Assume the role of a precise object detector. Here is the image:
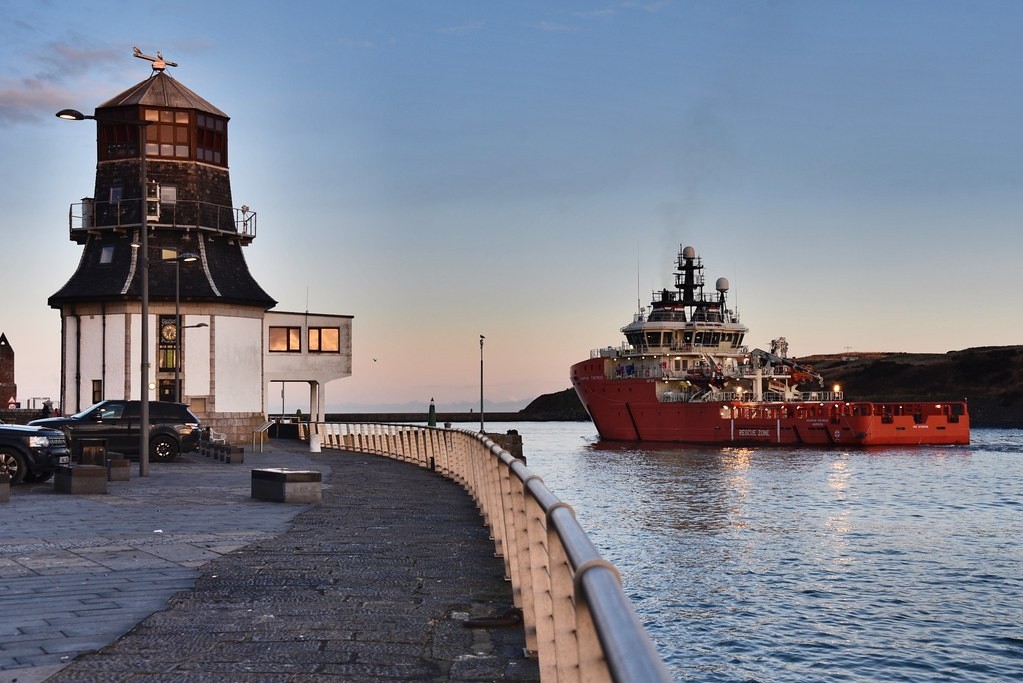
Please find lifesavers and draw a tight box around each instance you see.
[703,366,712,377]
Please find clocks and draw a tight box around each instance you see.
[162,324,176,341]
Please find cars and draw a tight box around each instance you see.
[0,418,73,484]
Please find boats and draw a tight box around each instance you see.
[571,243,971,449]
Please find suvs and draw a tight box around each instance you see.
[26,398,203,462]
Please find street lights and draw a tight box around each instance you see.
[54,105,152,476]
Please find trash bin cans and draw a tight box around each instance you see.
[76,437,109,467]
